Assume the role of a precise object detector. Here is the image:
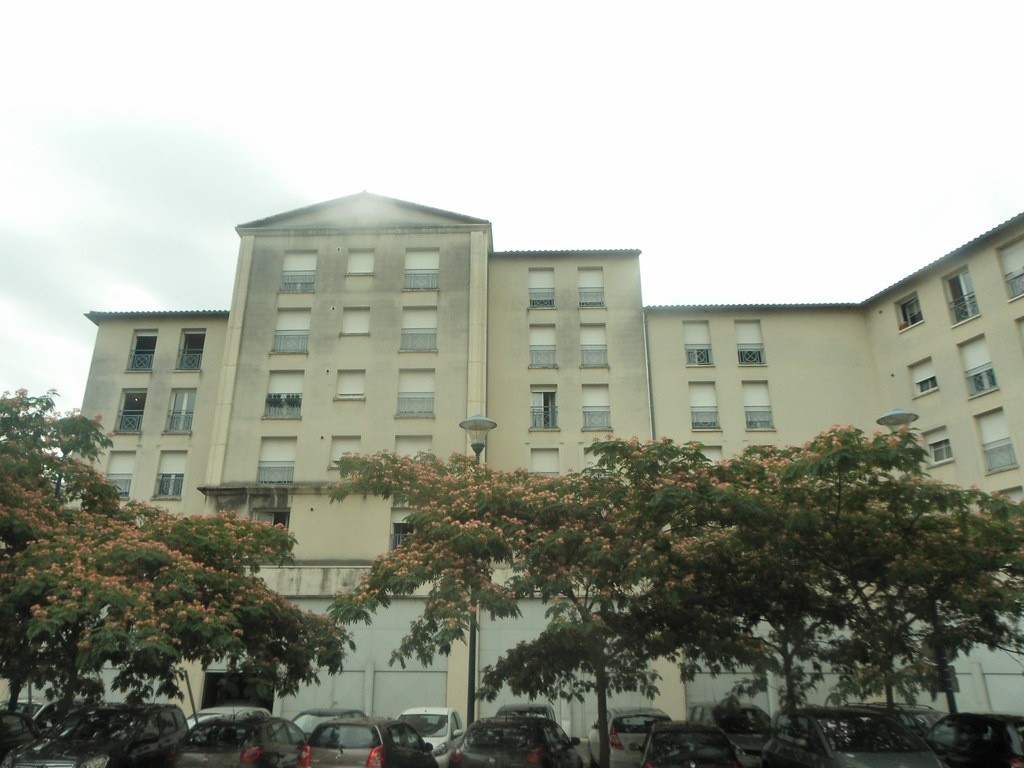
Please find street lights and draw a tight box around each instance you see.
[458,413,498,729]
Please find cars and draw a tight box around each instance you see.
[1,700,1024,768]
